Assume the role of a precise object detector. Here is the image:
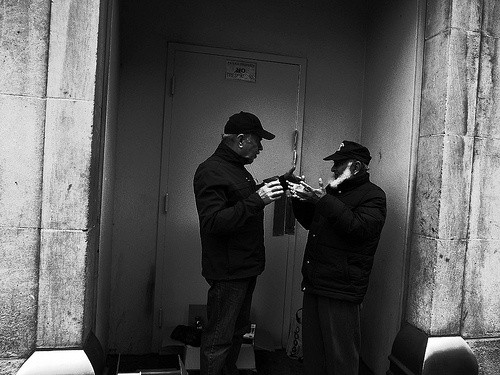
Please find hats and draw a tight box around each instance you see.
[224,111,275,140]
[323,141,372,165]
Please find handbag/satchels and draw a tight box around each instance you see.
[170,325,202,347]
[286,308,303,360]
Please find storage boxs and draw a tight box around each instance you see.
[161,304,256,370]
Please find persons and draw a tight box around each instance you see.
[286,140,387,375]
[193,110,306,375]
